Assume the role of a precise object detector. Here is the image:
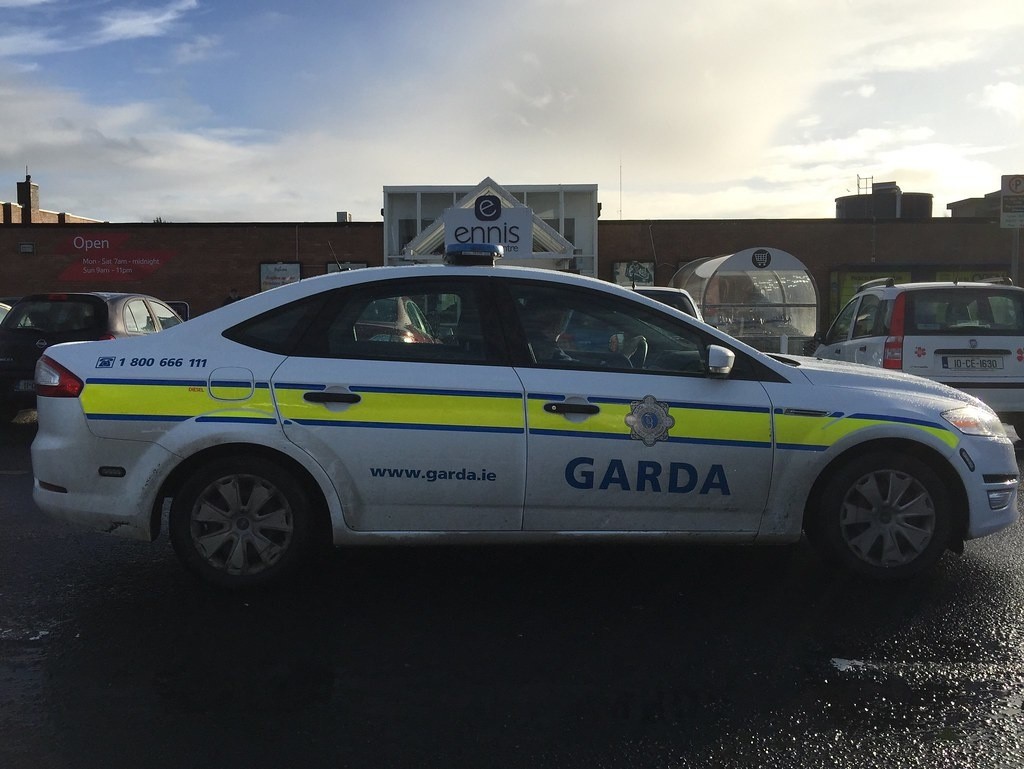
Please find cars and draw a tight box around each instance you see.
[816,274,1024,451]
[0,303,34,331]
[30,241,1019,597]
[353,296,455,346]
[0,290,190,437]
[543,285,722,377]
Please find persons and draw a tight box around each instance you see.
[525,293,647,363]
